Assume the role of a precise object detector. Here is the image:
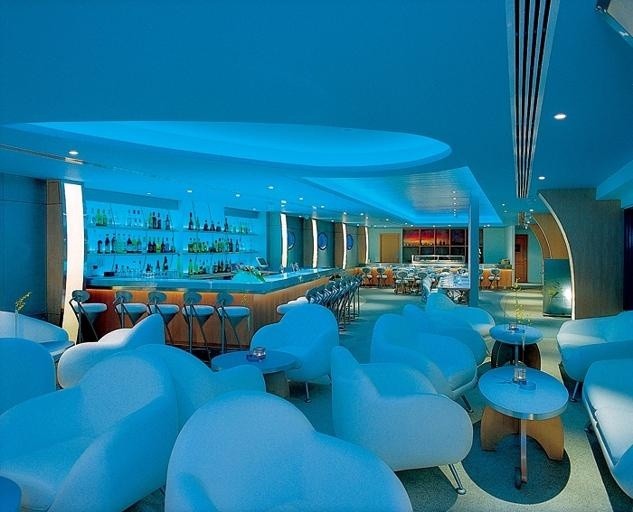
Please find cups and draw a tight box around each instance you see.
[513,367,526,384]
[508,322,516,330]
[252,347,266,358]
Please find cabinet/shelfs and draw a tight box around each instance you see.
[81,196,180,280]
[182,209,259,277]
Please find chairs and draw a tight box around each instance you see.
[357,262,502,302]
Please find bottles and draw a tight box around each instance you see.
[176,253,182,280]
[187,209,252,275]
[89,206,174,277]
